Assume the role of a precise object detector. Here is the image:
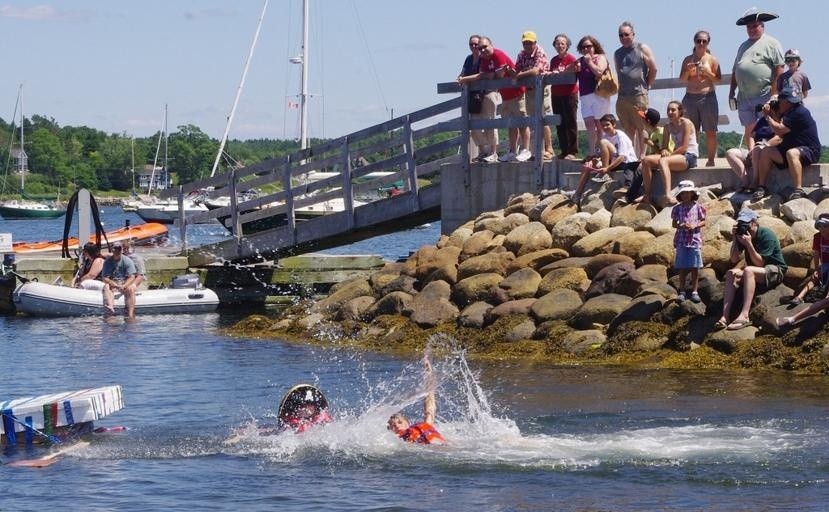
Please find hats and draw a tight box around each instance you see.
[638,108,661,124]
[521,31,537,44]
[736,7,780,26]
[736,209,759,223]
[814,213,829,230]
[670,179,700,202]
[776,87,802,105]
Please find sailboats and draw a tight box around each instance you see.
[205,0,393,237]
[0,83,66,220]
[137,99,284,224]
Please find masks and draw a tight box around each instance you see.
[783,49,801,60]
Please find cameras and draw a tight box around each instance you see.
[755,101,776,112]
[734,224,751,238]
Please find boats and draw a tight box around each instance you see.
[14,281,218,316]
[1,222,169,260]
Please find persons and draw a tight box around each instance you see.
[74,242,106,291]
[563,36,612,162]
[502,32,556,159]
[729,7,786,152]
[120,240,148,291]
[753,88,821,200]
[387,359,448,446]
[613,22,658,161]
[551,35,580,160]
[715,209,788,330]
[572,114,641,204]
[478,36,532,162]
[680,31,722,167]
[672,180,707,303]
[611,109,676,206]
[286,404,332,435]
[790,214,829,304]
[633,101,699,206]
[456,35,501,163]
[777,50,812,107]
[776,292,829,327]
[726,100,783,193]
[102,245,137,324]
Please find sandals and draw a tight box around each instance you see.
[564,153,578,159]
[750,185,770,200]
[544,149,556,161]
[676,290,687,301]
[689,291,702,303]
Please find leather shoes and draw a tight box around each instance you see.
[782,298,802,306]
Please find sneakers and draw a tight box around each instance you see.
[472,152,487,163]
[590,172,614,183]
[617,196,628,204]
[775,316,795,328]
[496,149,517,162]
[513,148,533,162]
[612,186,629,197]
[571,196,580,204]
[634,194,651,203]
[664,194,677,206]
[483,151,499,162]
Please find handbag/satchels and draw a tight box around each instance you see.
[594,54,618,99]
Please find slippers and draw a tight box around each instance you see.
[713,319,730,331]
[726,319,753,330]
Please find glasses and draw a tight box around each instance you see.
[580,45,594,50]
[696,39,708,44]
[523,42,534,46]
[785,58,797,63]
[478,45,489,50]
[468,43,479,46]
[619,32,632,37]
[746,23,761,28]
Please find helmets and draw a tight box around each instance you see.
[110,240,123,256]
